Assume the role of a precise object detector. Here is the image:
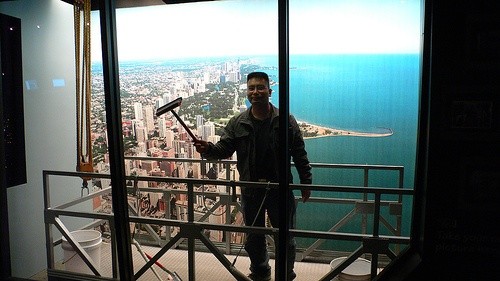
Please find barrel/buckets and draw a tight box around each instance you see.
[330,256,371,281]
[62,230,102,276]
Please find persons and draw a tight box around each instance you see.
[191,72,314,281]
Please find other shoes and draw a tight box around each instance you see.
[247,275,271,281]
[287,272,296,280]
